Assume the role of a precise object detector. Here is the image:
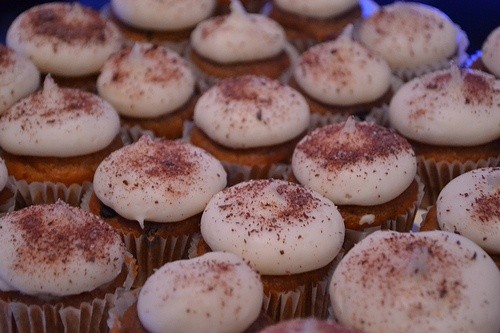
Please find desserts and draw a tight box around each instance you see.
[0,0,500,333]
